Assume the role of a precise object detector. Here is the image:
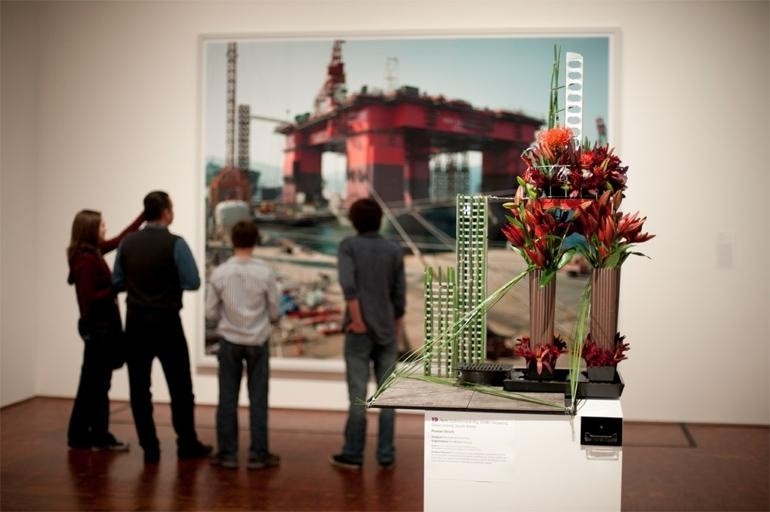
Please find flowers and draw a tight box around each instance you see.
[504,128,656,269]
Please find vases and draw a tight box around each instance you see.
[503,268,625,404]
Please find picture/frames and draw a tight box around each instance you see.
[194,29,621,373]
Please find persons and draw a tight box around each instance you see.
[204,220,283,471]
[107,190,213,465]
[328,198,407,473]
[65,209,147,449]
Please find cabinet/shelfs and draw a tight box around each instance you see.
[369,360,623,512]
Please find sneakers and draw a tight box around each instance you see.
[206,448,240,470]
[142,435,213,464]
[92,441,131,454]
[327,454,396,471]
[245,444,281,468]
